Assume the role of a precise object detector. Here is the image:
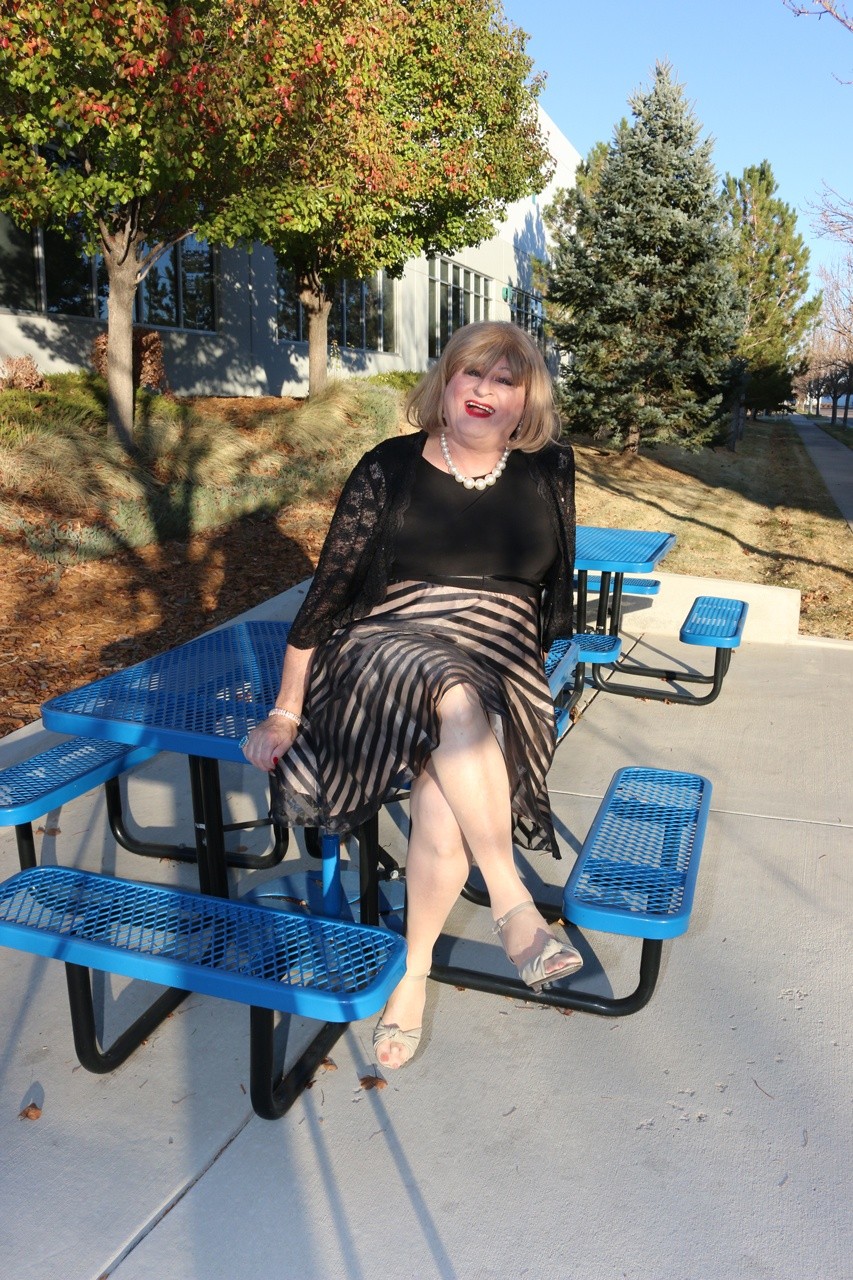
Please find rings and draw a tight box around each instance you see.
[239,736,249,749]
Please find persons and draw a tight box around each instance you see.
[239,321,586,1076]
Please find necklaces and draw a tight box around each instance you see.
[438,427,512,490]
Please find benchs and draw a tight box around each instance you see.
[0,864,408,1120]
[0,735,162,870]
[563,633,626,714]
[679,595,751,706]
[572,573,662,631]
[559,766,716,1018]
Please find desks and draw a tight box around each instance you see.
[37,617,582,973]
[561,524,677,689]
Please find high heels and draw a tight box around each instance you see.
[372,970,431,1070]
[491,901,584,988]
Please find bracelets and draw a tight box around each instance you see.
[268,709,303,727]
